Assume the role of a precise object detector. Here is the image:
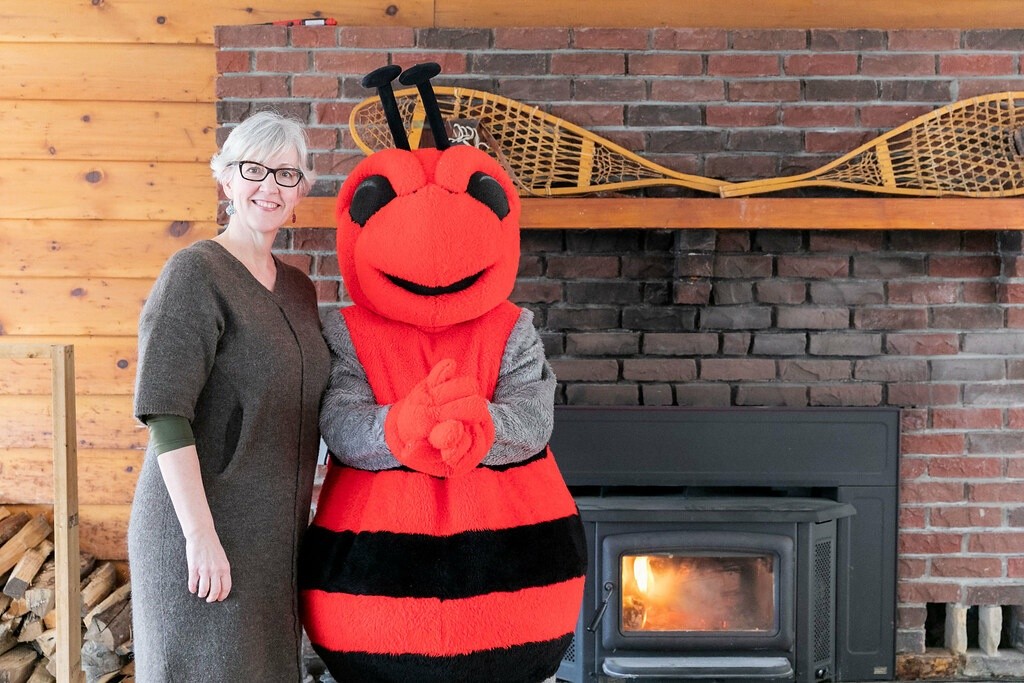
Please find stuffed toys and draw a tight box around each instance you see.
[297,58,583,683]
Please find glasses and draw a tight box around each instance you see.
[227,161,304,188]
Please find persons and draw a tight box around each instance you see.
[115,110,339,683]
